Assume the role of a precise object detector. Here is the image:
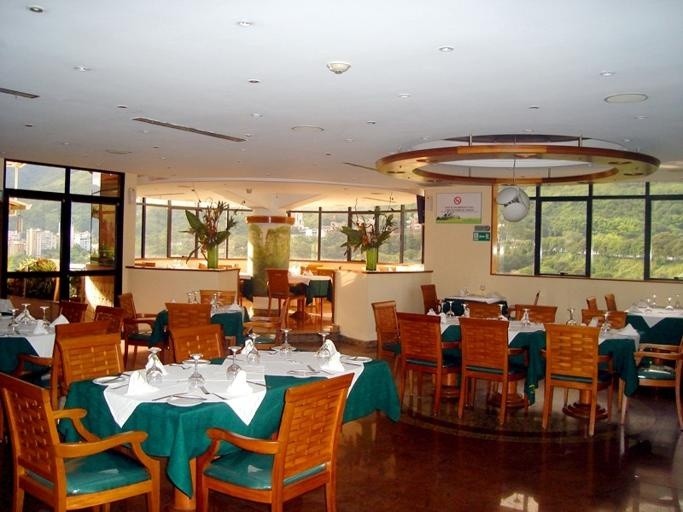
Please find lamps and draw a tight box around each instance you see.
[496,159,530,222]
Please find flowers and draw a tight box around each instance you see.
[178,190,246,264]
[340,195,399,256]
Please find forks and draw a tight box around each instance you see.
[246,382,271,389]
[201,386,227,400]
[306,364,332,377]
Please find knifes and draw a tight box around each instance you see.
[153,393,185,401]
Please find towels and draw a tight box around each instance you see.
[316,352,348,374]
[238,340,255,357]
[618,323,638,338]
[146,353,167,374]
[123,372,159,398]
[222,370,256,398]
[49,312,68,330]
[29,320,44,335]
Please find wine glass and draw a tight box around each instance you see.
[497,304,504,320]
[643,289,681,313]
[445,301,455,324]
[226,346,242,381]
[520,309,531,331]
[319,332,330,365]
[279,328,292,360]
[600,314,610,336]
[146,348,162,386]
[187,290,225,314]
[248,334,260,365]
[189,354,205,392]
[438,302,445,323]
[8,308,20,336]
[21,304,32,331]
[461,304,469,318]
[40,306,50,333]
[565,309,577,326]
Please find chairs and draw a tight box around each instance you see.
[200,289,236,306]
[507,291,540,318]
[458,318,528,426]
[582,309,628,413]
[28,299,60,323]
[605,294,617,310]
[170,324,225,363]
[300,264,322,313]
[586,296,598,310]
[494,304,558,399]
[119,293,156,368]
[59,300,88,323]
[55,332,124,409]
[93,305,125,329]
[621,337,683,430]
[465,303,501,400]
[8,295,30,316]
[196,372,354,512]
[165,303,213,329]
[371,301,404,378]
[396,312,462,417]
[421,284,441,315]
[0,373,160,512]
[542,322,614,437]
[317,267,338,323]
[243,298,289,350]
[17,319,111,425]
[265,268,305,329]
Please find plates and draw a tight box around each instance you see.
[287,369,312,378]
[184,359,211,366]
[272,345,297,351]
[168,396,203,407]
[351,356,374,363]
[92,376,126,387]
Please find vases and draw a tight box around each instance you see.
[366,247,377,270]
[208,243,218,268]
